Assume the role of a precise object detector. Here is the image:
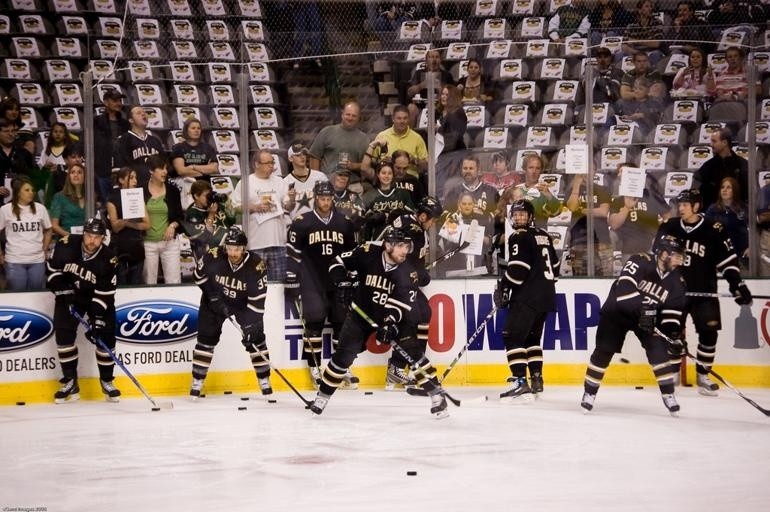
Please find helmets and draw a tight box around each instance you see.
[511,199,534,212]
[677,188,702,203]
[314,181,335,195]
[653,232,685,253]
[84,218,107,236]
[383,226,413,242]
[225,228,247,246]
[418,196,443,218]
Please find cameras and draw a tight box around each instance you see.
[207,192,228,206]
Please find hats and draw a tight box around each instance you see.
[103,89,127,99]
[596,47,612,56]
[287,144,310,157]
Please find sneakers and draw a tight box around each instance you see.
[581,391,596,410]
[500,377,531,397]
[310,366,322,383]
[258,375,272,395]
[661,393,680,411]
[697,372,719,390]
[191,376,204,396]
[100,376,120,397]
[531,376,543,393]
[310,392,330,414]
[344,369,359,383]
[431,391,447,413]
[386,364,411,381]
[55,376,79,398]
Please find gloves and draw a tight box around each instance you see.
[336,284,361,312]
[241,323,261,347]
[637,313,655,337]
[666,332,688,357]
[85,313,107,344]
[494,285,511,309]
[284,282,304,304]
[730,276,752,304]
[64,294,78,321]
[375,320,400,344]
[207,290,231,317]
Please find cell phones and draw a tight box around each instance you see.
[538,179,544,185]
[289,181,296,191]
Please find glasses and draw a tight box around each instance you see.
[261,161,275,165]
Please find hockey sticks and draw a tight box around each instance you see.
[65,303,173,410]
[352,300,486,409]
[293,301,360,384]
[654,326,770,417]
[224,310,315,406]
[407,304,499,396]
[426,219,478,269]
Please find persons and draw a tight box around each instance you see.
[652,190,752,397]
[455,58,498,115]
[386,196,444,392]
[0,91,770,291]
[546,1,769,91]
[579,234,689,418]
[309,227,451,420]
[407,51,454,130]
[291,0,327,70]
[418,1,458,27]
[434,83,471,154]
[188,230,277,395]
[285,183,359,390]
[492,199,561,399]
[370,1,433,32]
[46,218,124,403]
